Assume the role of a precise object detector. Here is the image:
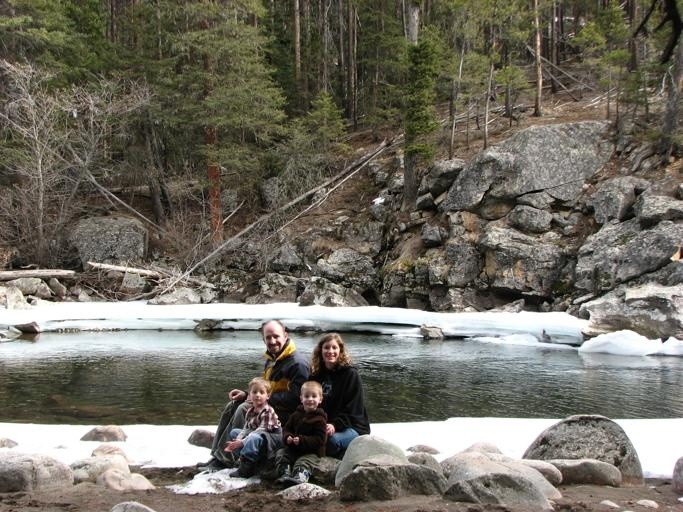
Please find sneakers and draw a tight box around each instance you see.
[197,458,217,467]
[198,460,224,472]
[287,466,310,484]
[276,463,291,483]
[230,461,255,478]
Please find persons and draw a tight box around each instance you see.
[311,333,371,459]
[275,380,327,484]
[197,318,310,473]
[224,377,283,478]
